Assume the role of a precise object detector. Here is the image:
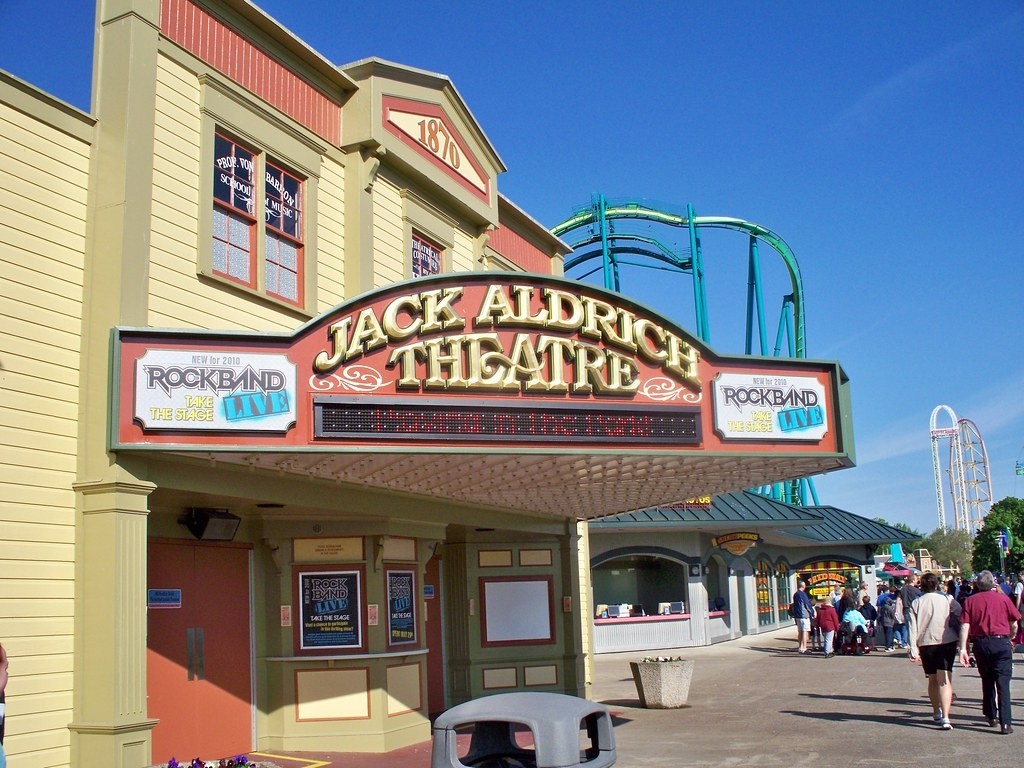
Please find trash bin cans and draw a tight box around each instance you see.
[431,690,617,768]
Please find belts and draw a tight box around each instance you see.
[975,635,1006,640]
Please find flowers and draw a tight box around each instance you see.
[640,655,684,662]
[159,754,256,768]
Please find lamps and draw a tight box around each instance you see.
[865,566,872,574]
[727,567,734,577]
[702,566,709,576]
[175,507,241,541]
[753,567,758,577]
[774,570,778,577]
[688,565,700,577]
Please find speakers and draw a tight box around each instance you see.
[185,510,242,541]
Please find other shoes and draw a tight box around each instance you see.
[799,648,812,655]
[941,720,952,729]
[932,710,942,723]
[825,653,834,658]
[989,717,999,727]
[884,648,894,652]
[1000,723,1013,734]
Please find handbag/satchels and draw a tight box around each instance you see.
[947,612,960,632]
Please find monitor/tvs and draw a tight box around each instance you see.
[715,598,725,607]
[657,602,683,614]
[596,605,643,617]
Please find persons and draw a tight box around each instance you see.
[957,570,1022,735]
[905,573,964,729]
[792,568,1023,659]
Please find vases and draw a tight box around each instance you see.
[147,759,280,768]
[629,658,695,709]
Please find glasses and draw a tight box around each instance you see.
[1018,574,1024,578]
